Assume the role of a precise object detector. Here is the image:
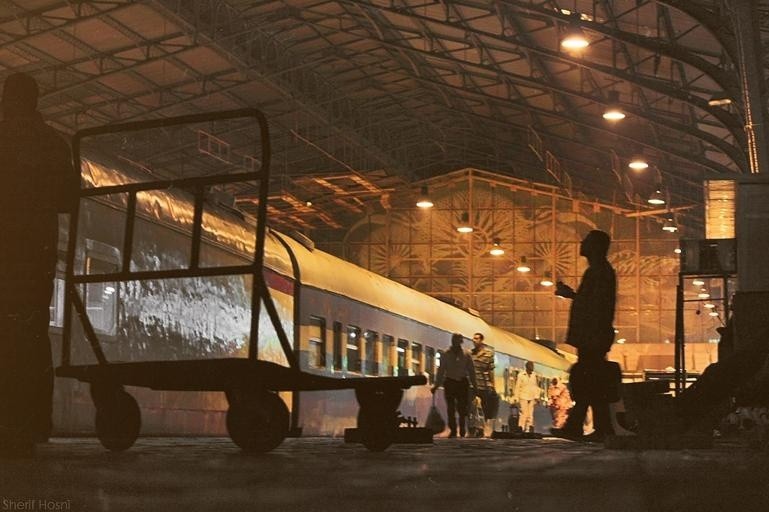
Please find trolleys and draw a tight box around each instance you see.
[54,106,427,455]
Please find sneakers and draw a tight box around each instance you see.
[549,427,585,443]
[585,429,617,444]
[425,406,445,434]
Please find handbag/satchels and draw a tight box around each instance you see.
[569,360,621,403]
[487,392,500,419]
[468,396,485,431]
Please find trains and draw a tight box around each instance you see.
[49,154,593,438]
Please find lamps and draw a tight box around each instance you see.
[646,179,677,231]
[414,154,554,288]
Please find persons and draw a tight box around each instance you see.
[549,377,575,429]
[466,332,495,439]
[547,230,624,445]
[429,332,478,438]
[513,360,540,434]
